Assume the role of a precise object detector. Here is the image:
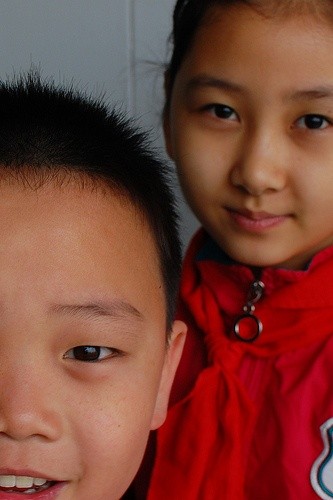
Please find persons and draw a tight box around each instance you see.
[119,0,333,500]
[0,74,187,500]
